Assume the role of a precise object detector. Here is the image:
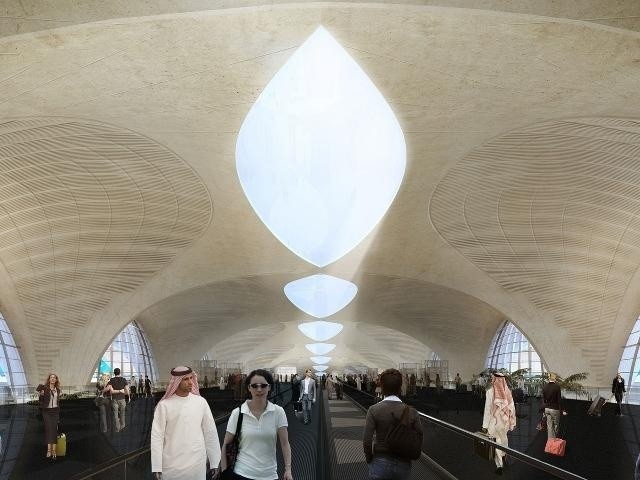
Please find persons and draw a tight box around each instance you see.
[129,375,137,401]
[471,371,477,398]
[517,374,524,391]
[435,373,440,388]
[424,375,431,391]
[221,370,294,480]
[363,368,424,479]
[137,374,144,400]
[148,364,222,479]
[482,372,517,476]
[95,372,125,433]
[478,373,485,398]
[455,372,462,392]
[202,375,209,390]
[142,375,153,400]
[611,369,626,416]
[39,373,61,459]
[97,368,131,433]
[542,372,569,442]
[299,368,317,426]
[220,372,417,415]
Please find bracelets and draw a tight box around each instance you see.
[284,463,291,468]
